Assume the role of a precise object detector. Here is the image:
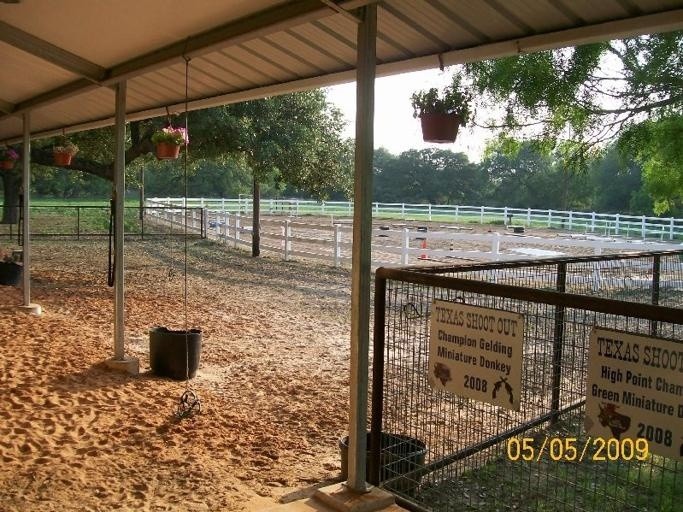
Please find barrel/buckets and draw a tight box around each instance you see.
[149,326,204,378]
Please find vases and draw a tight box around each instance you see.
[0,160,14,169]
[51,153,72,166]
[156,143,180,159]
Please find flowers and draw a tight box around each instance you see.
[0,146,19,162]
[151,125,189,147]
[53,142,80,157]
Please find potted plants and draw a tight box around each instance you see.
[411,75,474,143]
